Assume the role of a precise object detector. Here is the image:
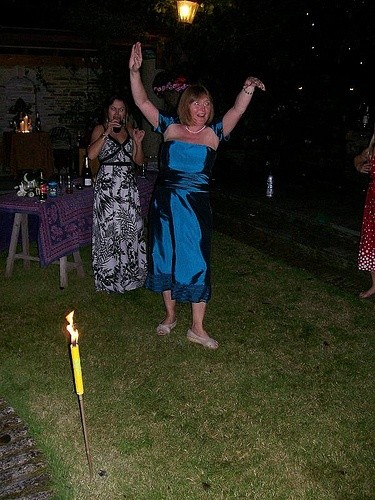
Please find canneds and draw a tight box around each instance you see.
[48,182,58,198]
[28,187,35,198]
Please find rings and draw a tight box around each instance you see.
[259,81,262,83]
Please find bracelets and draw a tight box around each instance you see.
[244,89,253,95]
[100,132,107,140]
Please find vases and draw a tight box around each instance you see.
[162,91,182,108]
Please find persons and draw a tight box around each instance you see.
[87,95,148,292]
[129,42,265,350]
[18,112,33,133]
[353,131,375,299]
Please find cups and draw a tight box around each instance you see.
[48,183,58,198]
[56,174,65,196]
[64,175,74,194]
[113,114,121,133]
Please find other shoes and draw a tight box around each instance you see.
[187,328,220,350]
[156,316,178,335]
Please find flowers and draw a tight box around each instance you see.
[152,81,191,95]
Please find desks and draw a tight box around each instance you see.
[0,170,159,290]
[3,132,52,178]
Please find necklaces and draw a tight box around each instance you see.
[184,125,206,134]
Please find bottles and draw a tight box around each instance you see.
[76,130,82,147]
[264,172,275,201]
[358,102,370,137]
[83,156,92,187]
[141,162,147,178]
[34,111,41,132]
[38,171,47,201]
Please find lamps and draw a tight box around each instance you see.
[176,0,200,25]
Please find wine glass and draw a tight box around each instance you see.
[9,120,17,135]
